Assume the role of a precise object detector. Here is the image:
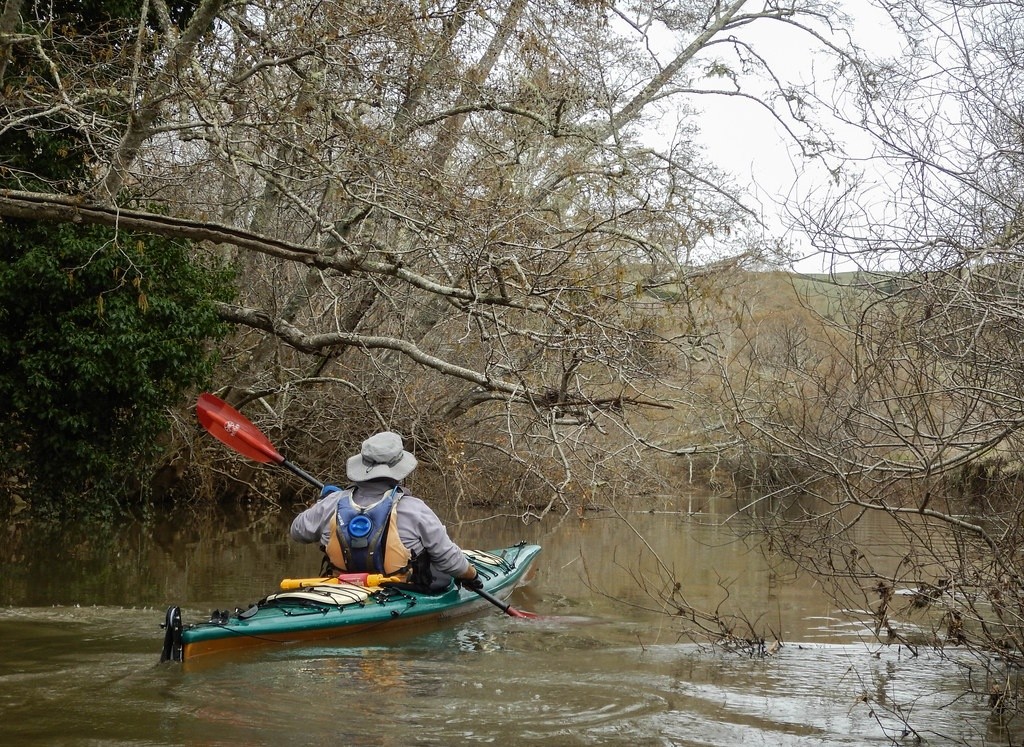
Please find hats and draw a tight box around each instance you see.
[347,431,418,482]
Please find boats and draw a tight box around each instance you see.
[160,539,542,664]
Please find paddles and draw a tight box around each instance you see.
[195,392,535,617]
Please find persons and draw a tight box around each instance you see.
[291,432,484,595]
